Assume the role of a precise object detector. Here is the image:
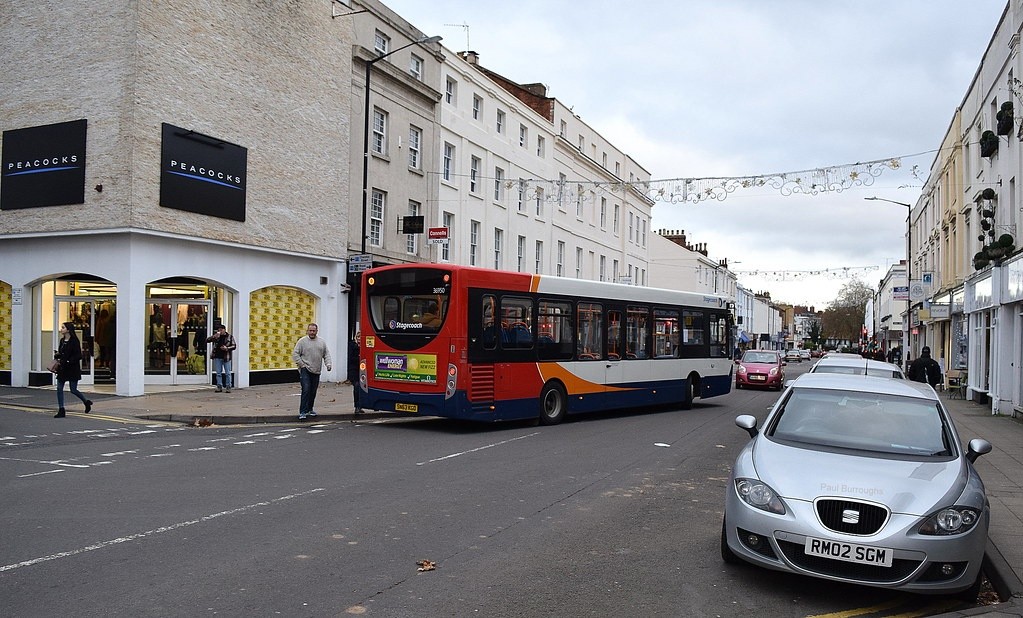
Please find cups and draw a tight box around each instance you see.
[217,332,220,337]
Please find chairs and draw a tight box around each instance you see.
[946,369,970,400]
[483,319,637,361]
[747,355,777,362]
[800,398,929,439]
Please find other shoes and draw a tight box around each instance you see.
[215,386,222,393]
[226,385,232,393]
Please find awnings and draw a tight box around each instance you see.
[741,331,753,342]
[867,340,873,348]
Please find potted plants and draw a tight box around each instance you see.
[995,102,1013,136]
[979,131,999,158]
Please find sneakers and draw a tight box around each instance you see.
[306,410,317,416]
[299,412,306,420]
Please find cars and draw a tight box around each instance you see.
[721,372,994,602]
[735,350,786,392]
[786,350,802,362]
[776,350,788,362]
[810,351,820,358]
[811,355,907,381]
[799,350,811,361]
[822,352,862,359]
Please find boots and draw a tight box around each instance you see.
[84,400,93,413]
[54,407,66,418]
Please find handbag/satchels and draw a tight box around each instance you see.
[47,358,61,374]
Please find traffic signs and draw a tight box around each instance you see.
[349,262,373,273]
[349,253,373,264]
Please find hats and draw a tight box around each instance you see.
[922,346,930,354]
[218,325,225,329]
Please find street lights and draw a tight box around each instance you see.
[362,33,444,254]
[864,288,876,360]
[863,195,912,377]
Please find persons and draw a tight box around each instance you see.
[865,348,894,363]
[150,313,167,367]
[422,303,441,327]
[908,346,941,390]
[80,310,111,368]
[630,342,639,359]
[54,323,93,418]
[293,324,332,419]
[348,332,364,414]
[206,325,236,393]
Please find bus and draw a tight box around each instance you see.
[359,263,739,427]
